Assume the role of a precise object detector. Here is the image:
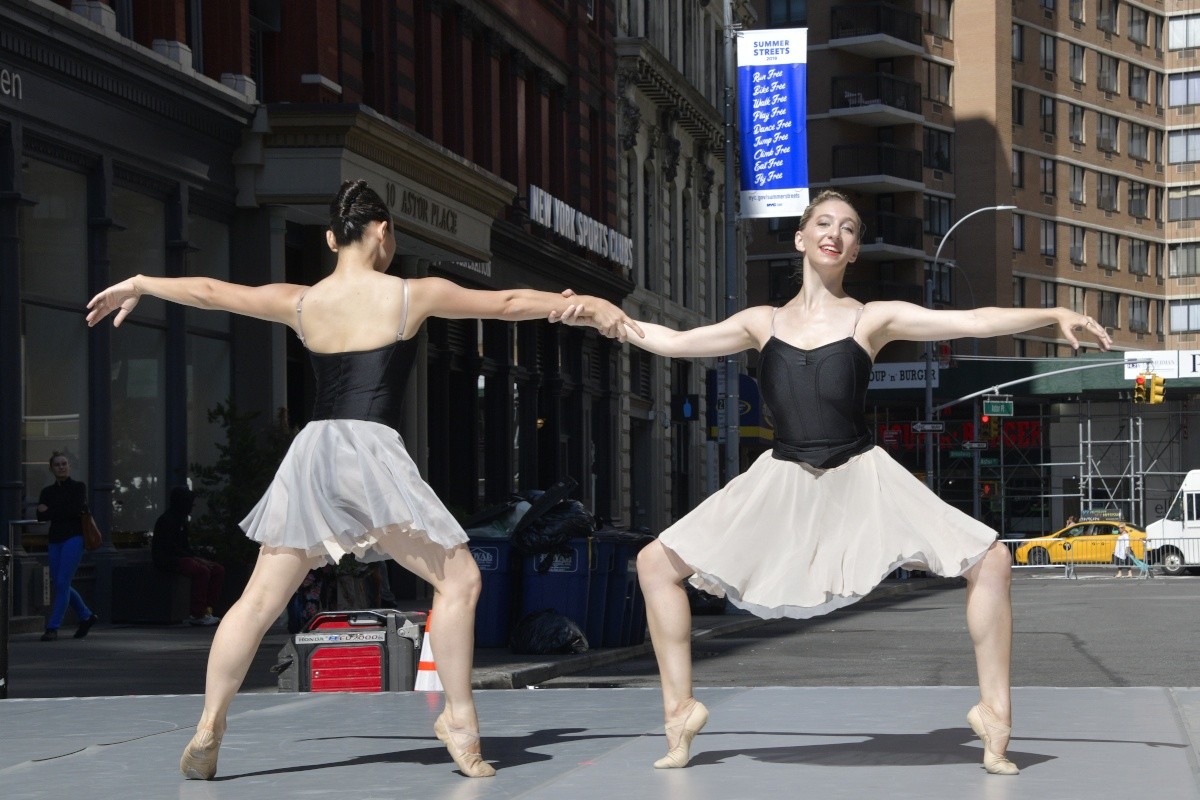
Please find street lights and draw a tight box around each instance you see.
[943,262,981,521]
[926,205,1019,487]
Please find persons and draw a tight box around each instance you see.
[547,191,1112,777]
[1065,516,1078,528]
[152,486,224,625]
[36,451,103,641]
[86,178,646,780]
[1114,521,1134,577]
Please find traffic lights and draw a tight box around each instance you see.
[984,481,997,495]
[990,416,1000,442]
[981,414,990,443]
[1150,374,1166,405]
[1134,375,1147,404]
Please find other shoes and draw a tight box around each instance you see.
[74,613,98,638]
[189,614,219,626]
[41,628,57,641]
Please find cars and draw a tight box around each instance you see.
[1015,507,1151,566]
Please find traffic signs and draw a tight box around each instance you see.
[910,422,945,434]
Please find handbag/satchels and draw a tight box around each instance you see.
[534,508,575,558]
[80,511,102,551]
[511,608,589,653]
[556,500,597,535]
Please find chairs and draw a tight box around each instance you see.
[843,90,871,108]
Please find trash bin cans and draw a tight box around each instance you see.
[609,526,657,647]
[461,534,517,650]
[272,606,427,694]
[520,528,617,648]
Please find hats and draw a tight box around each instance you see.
[1118,525,1124,528]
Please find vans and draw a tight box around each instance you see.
[1143,469,1200,576]
